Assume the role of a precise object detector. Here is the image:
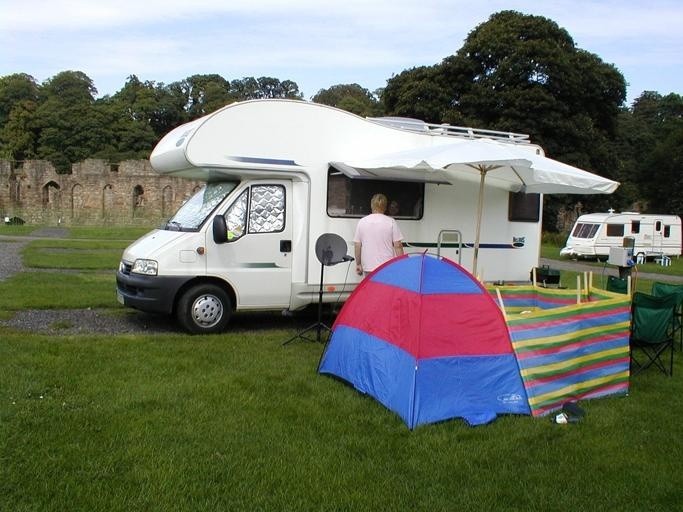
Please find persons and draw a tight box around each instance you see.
[353,193,405,278]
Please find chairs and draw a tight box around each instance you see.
[605,275,683,378]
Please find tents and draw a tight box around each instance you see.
[318,253,532,432]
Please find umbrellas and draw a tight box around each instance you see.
[328,137,623,282]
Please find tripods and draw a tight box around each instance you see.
[282,267,330,348]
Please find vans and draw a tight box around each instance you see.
[558,209,682,260]
[115,98,546,334]
